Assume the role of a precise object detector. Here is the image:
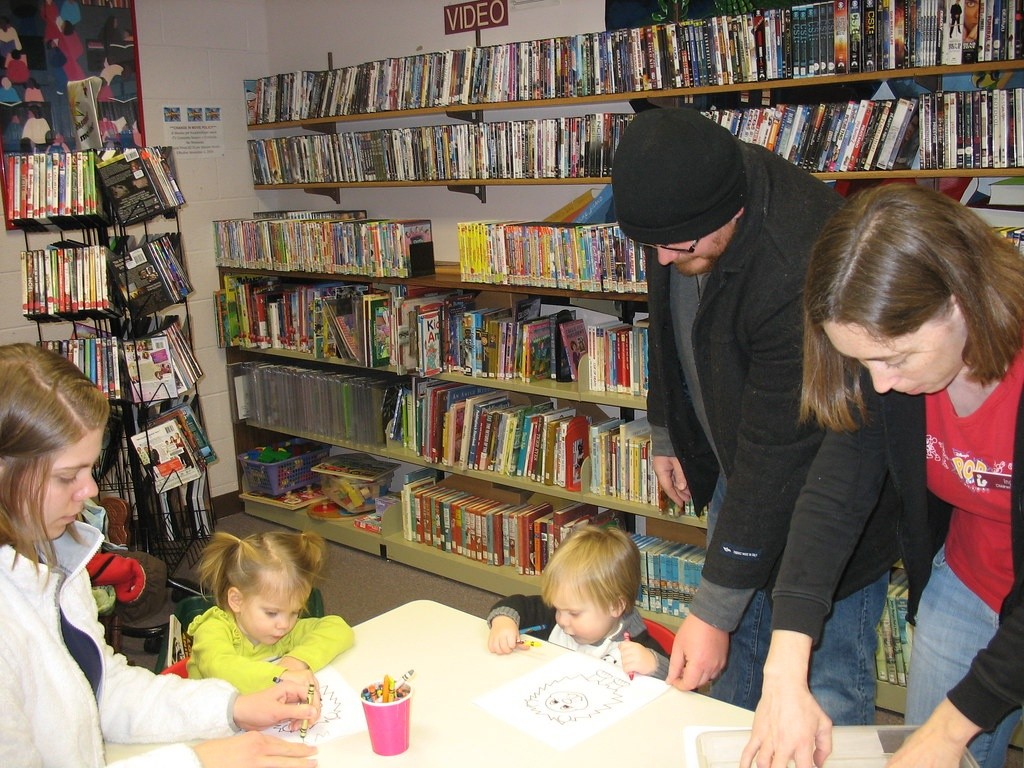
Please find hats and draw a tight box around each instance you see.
[611,106,749,246]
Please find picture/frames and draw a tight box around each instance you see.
[0,0,148,229]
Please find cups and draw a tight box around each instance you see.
[362,682,411,757]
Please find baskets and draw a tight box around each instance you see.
[237,438,332,496]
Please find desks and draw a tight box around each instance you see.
[101,598,982,766]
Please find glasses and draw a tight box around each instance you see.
[635,238,701,253]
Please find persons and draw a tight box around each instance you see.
[612,105,896,727]
[187,525,356,698]
[736,175,1022,768]
[885,396,1023,768]
[0,342,323,766]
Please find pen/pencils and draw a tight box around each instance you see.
[360,668,414,705]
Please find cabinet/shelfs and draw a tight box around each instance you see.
[2,142,218,557]
[209,2,1024,749]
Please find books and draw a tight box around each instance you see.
[214,0,1023,686]
[0,144,224,539]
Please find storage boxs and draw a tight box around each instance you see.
[236,436,329,495]
[316,459,395,514]
